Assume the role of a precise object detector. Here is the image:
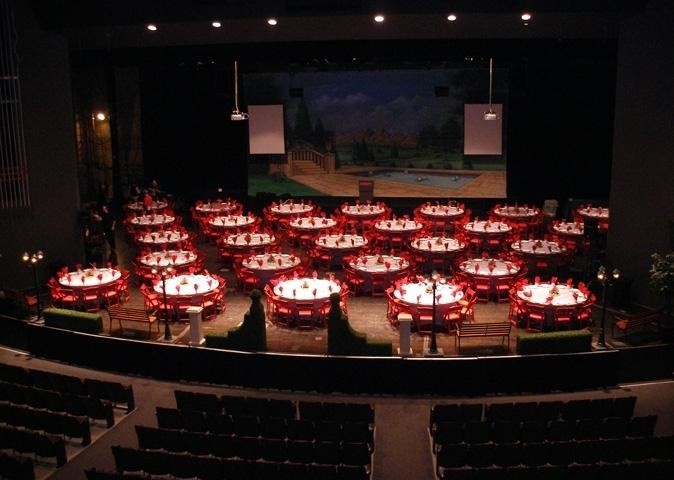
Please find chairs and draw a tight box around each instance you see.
[423,397,669,475]
[384,253,597,333]
[49,261,130,313]
[140,268,226,321]
[123,196,608,298]
[88,390,377,477]
[263,269,350,330]
[1,363,135,478]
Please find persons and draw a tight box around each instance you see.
[128,178,160,201]
[88,213,103,235]
[101,204,118,253]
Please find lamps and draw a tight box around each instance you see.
[483,58,499,122]
[230,62,249,122]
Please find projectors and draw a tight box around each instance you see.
[484,113,496,120]
[231,113,250,121]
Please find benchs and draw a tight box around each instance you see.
[453,319,512,353]
[105,305,159,337]
[610,306,667,342]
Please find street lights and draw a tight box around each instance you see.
[151,257,176,340]
[422,270,442,354]
[597,265,620,347]
[21,251,44,321]
